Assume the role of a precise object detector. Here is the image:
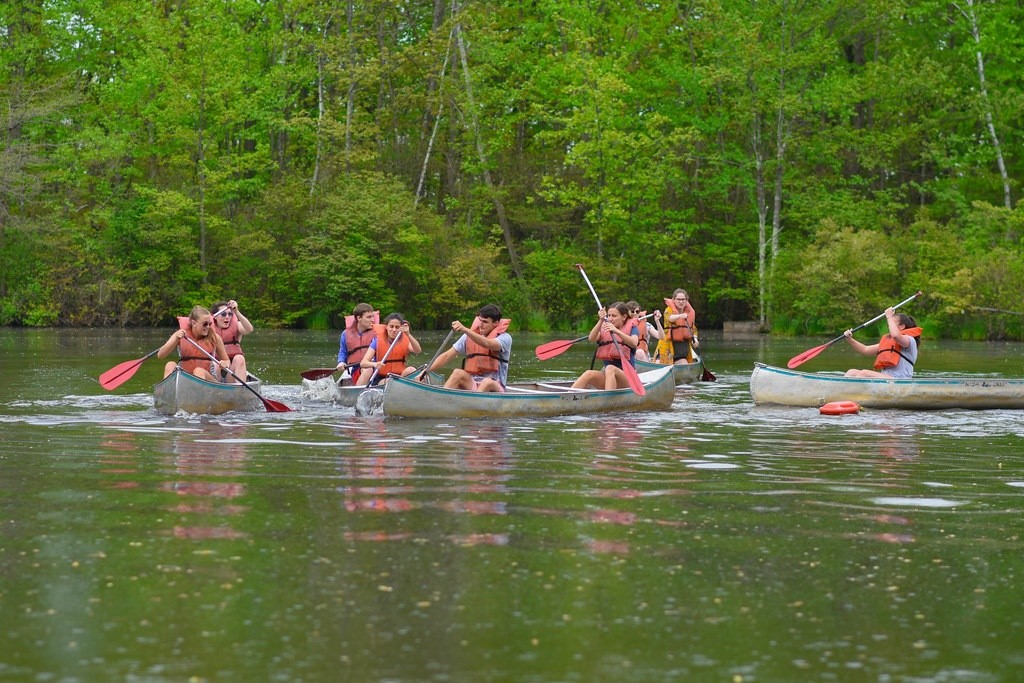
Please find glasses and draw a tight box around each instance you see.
[197,321,213,326]
[220,312,233,316]
[631,310,640,315]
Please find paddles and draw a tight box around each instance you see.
[183,334,292,412]
[365,331,402,390]
[300,358,376,381]
[535,312,656,361]
[787,290,923,369]
[575,263,646,396]
[99,305,231,391]
[419,328,456,381]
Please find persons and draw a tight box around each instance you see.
[164,305,231,382]
[336,303,374,386]
[360,313,421,385]
[415,303,512,393]
[843,307,921,378]
[567,302,640,392]
[211,300,253,382]
[627,288,699,364]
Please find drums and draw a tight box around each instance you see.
[685,317,716,381]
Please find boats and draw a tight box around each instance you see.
[383,364,675,418]
[153,366,262,417]
[631,357,702,383]
[336,364,446,405]
[749,360,1024,408]
